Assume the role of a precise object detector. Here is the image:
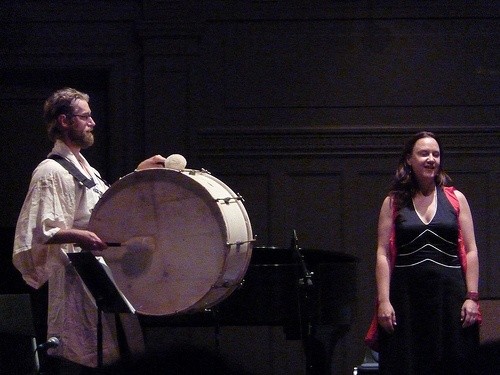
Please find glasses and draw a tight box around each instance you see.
[66,113,93,120]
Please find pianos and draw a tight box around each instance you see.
[0,245,361,375]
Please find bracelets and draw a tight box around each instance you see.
[466,291,479,301]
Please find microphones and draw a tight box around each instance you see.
[37,336,60,351]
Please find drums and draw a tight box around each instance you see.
[84,164,256,317]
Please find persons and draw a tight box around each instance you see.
[13,88,166,367]
[376,132,482,375]
